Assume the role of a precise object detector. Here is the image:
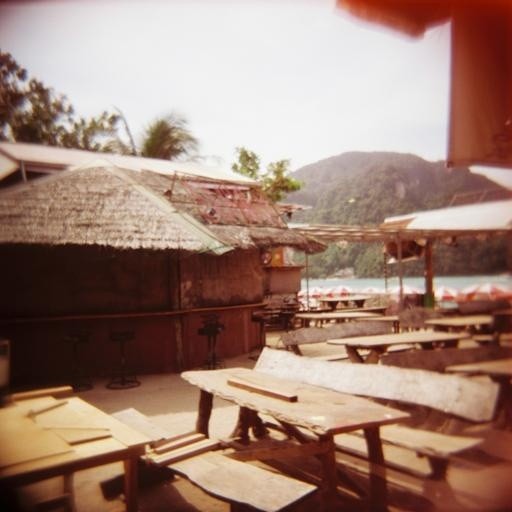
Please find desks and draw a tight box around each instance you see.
[325,329,470,365]
[0,385,150,512]
[180,365,412,511]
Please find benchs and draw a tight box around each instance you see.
[99,408,318,511]
[279,322,416,362]
[377,346,511,374]
[249,347,501,510]
